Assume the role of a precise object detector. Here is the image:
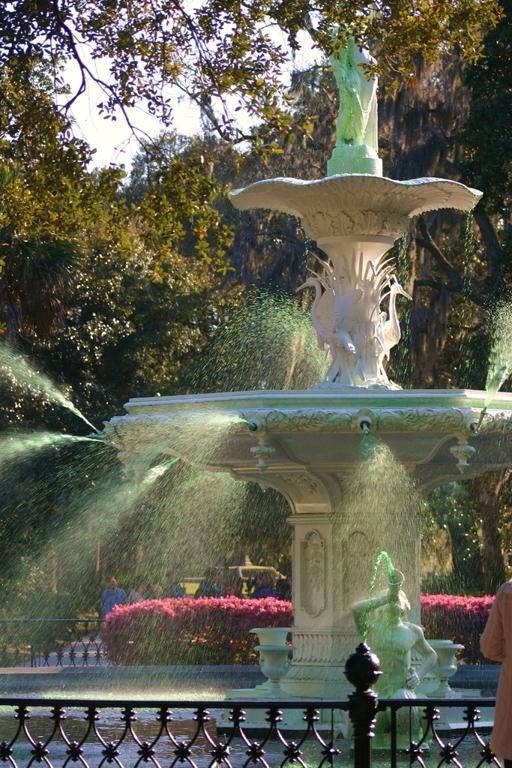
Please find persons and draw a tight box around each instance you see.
[480,580,512,768]
[351,589,438,750]
[100,567,292,616]
